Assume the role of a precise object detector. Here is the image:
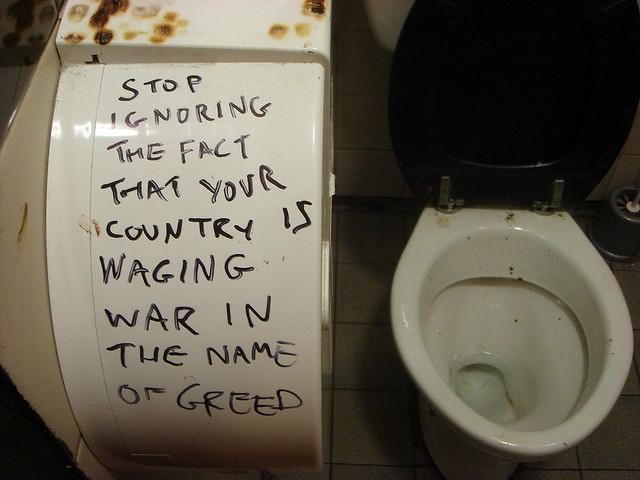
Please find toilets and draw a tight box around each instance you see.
[389,0,640,479]
